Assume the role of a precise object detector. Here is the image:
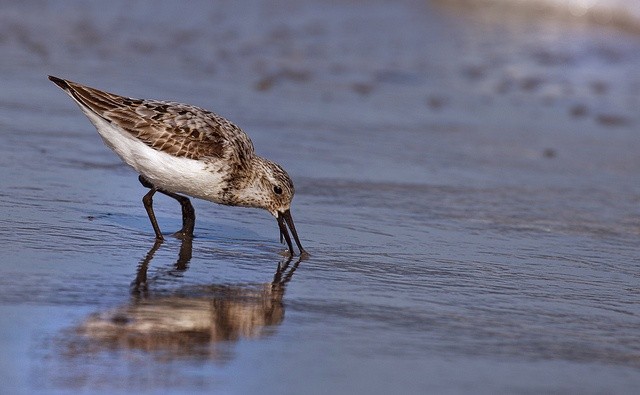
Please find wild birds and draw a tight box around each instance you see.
[48,75,308,257]
